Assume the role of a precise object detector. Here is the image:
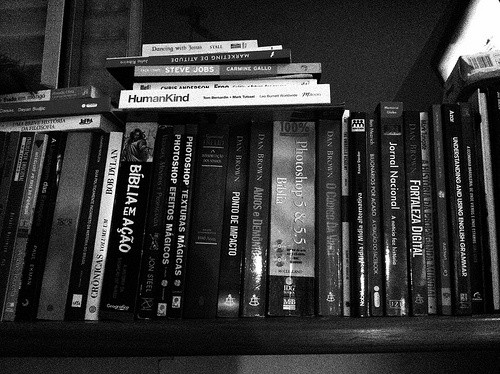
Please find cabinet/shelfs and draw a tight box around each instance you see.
[0,0,500,374]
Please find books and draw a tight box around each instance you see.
[0,38,500,322]
